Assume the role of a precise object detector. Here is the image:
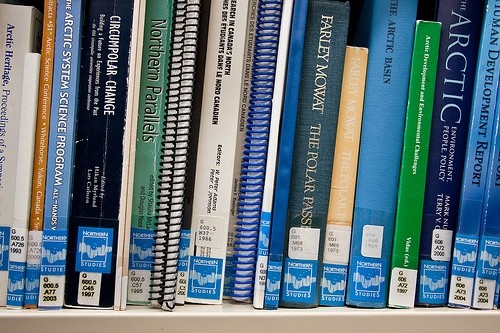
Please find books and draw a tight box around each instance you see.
[0,0,500,311]
